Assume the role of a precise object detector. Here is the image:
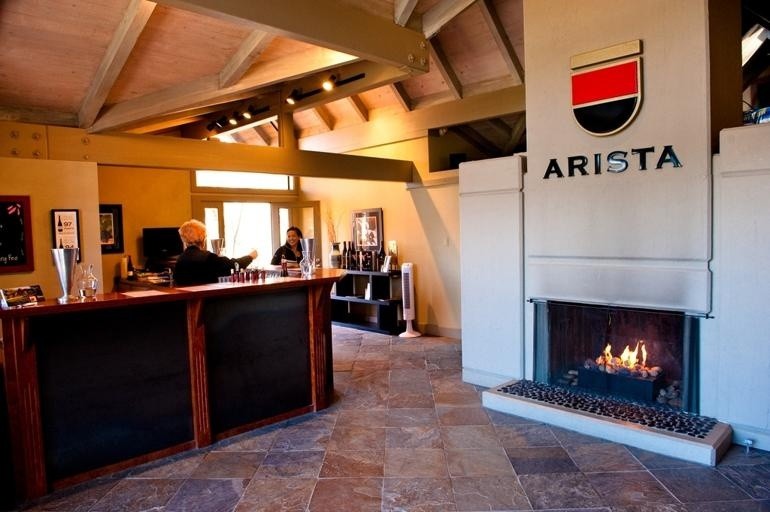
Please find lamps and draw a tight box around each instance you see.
[215,115,226,127]
[322,74,337,91]
[242,105,253,119]
[229,111,239,127]
[285,89,298,106]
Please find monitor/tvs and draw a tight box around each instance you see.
[143,227,183,262]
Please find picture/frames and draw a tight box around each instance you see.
[51,209,81,265]
[0,192,36,274]
[97,203,125,255]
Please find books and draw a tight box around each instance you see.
[120,258,173,284]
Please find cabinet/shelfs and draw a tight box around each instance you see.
[342,243,403,335]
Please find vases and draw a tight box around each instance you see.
[330,242,342,268]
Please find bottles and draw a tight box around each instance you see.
[280,254,288,277]
[228,261,265,283]
[342,240,386,271]
[299,250,317,276]
[127,255,135,281]
[78,263,100,299]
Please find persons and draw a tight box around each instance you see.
[172,219,257,286]
[270,227,305,268]
